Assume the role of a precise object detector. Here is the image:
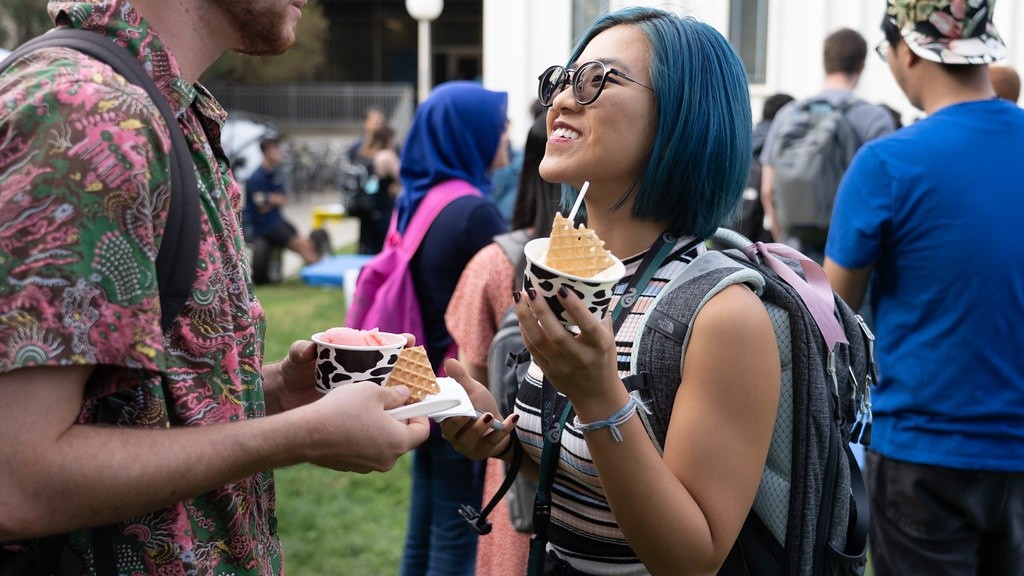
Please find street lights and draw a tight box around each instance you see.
[405,0,444,109]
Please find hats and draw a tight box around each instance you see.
[886,0,1009,64]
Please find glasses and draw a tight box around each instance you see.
[875,30,901,64]
[538,61,656,107]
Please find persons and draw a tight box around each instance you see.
[246,130,320,264]
[339,106,401,256]
[391,81,511,576]
[0,0,430,576]
[738,92,796,240]
[444,7,779,576]
[988,63,1021,102]
[822,0,1024,576]
[441,100,589,576]
[745,26,902,471]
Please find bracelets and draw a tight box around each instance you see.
[573,395,652,443]
[263,193,268,205]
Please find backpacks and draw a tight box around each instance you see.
[343,177,482,447]
[488,229,540,533]
[769,98,870,248]
[629,228,878,576]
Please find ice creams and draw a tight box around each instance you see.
[316,325,391,347]
[539,249,621,280]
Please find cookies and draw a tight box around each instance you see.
[385,344,442,406]
[543,211,616,278]
